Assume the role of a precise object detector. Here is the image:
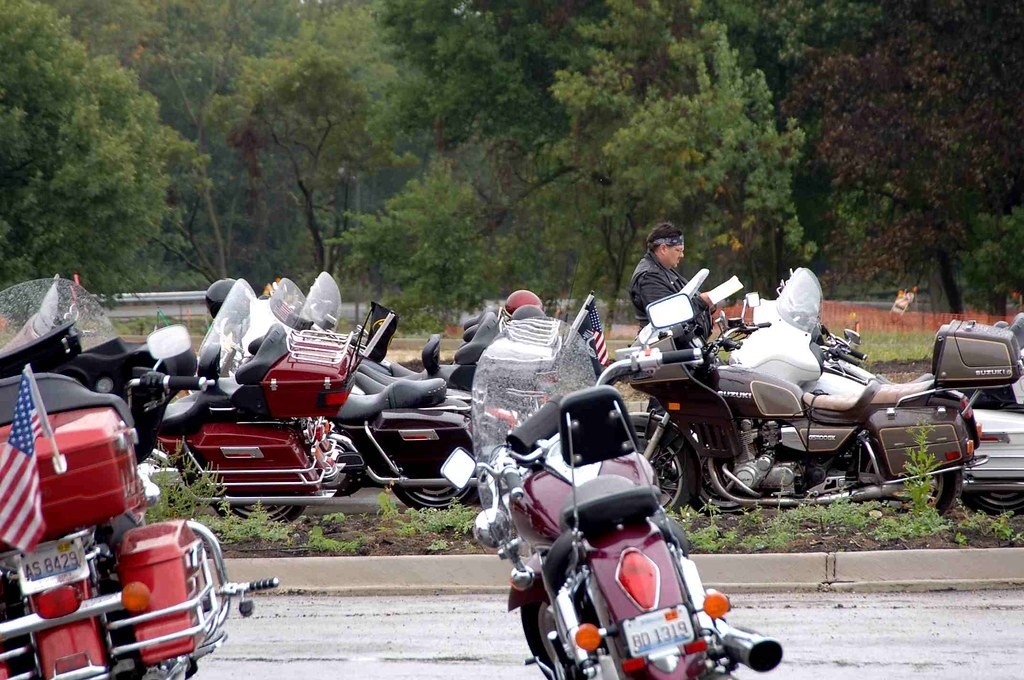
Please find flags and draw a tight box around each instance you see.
[0,370,52,553]
[588,303,608,365]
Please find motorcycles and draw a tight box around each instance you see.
[590,292,1024,517]
[124,277,478,514]
[624,266,1023,517]
[0,278,279,680]
[439,317,785,679]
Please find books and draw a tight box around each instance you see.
[710,275,743,303]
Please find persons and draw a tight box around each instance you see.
[630,223,716,417]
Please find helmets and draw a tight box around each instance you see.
[205,277,250,321]
[505,289,545,315]
[510,304,546,321]
